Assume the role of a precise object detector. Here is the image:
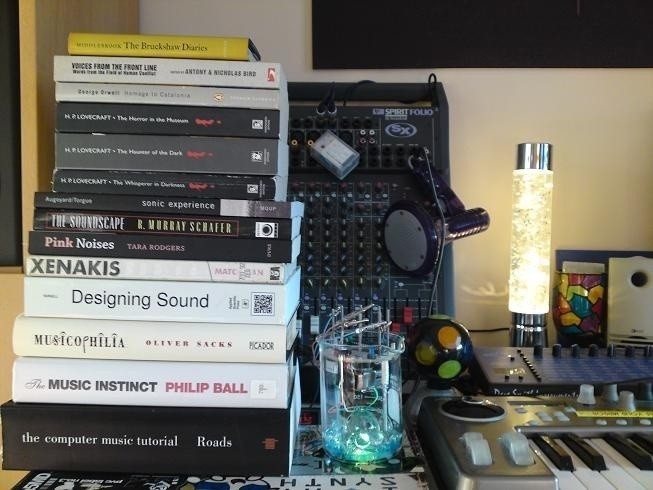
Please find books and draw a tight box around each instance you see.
[1,31,303,480]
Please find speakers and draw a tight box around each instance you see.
[607,256,653,350]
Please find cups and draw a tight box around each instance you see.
[316,331,405,463]
[553,269,606,338]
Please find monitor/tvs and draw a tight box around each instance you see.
[312,1,653,69]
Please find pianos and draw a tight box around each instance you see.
[417,394,652,489]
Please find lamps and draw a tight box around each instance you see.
[509,142,553,345]
